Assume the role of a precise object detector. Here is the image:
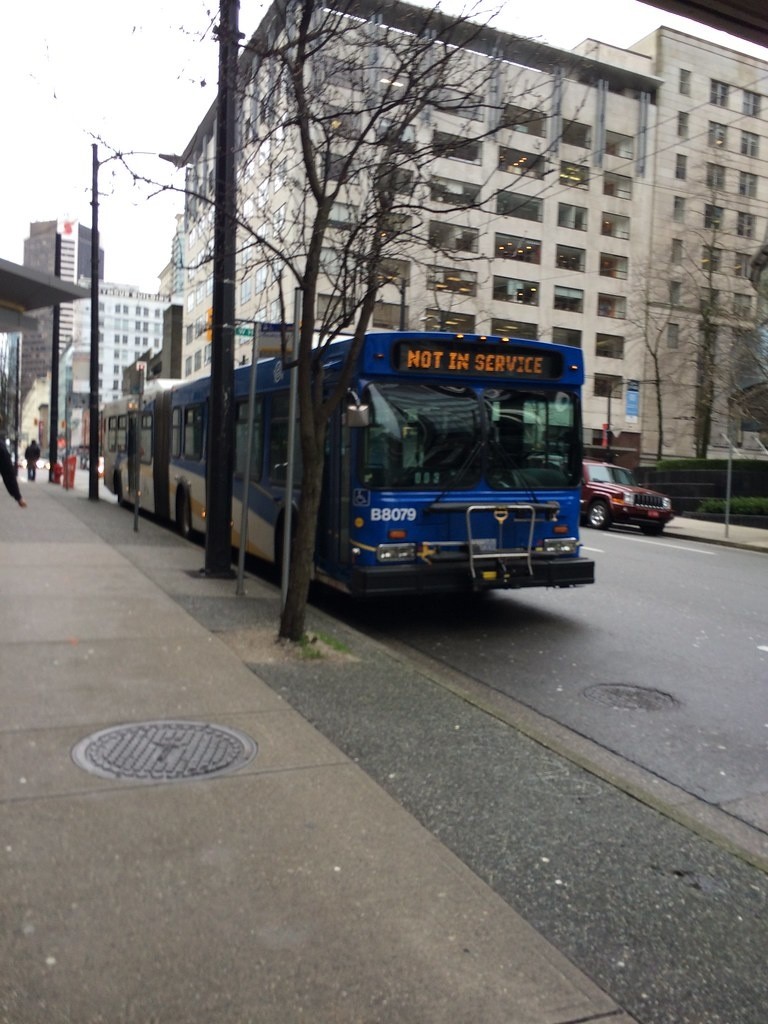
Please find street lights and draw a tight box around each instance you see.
[89,142,187,501]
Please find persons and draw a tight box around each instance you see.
[0,442,27,507]
[25,440,40,481]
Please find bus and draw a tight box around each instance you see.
[103,332,595,600]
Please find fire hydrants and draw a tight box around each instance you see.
[53,464,62,483]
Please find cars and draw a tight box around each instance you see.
[580,459,674,536]
